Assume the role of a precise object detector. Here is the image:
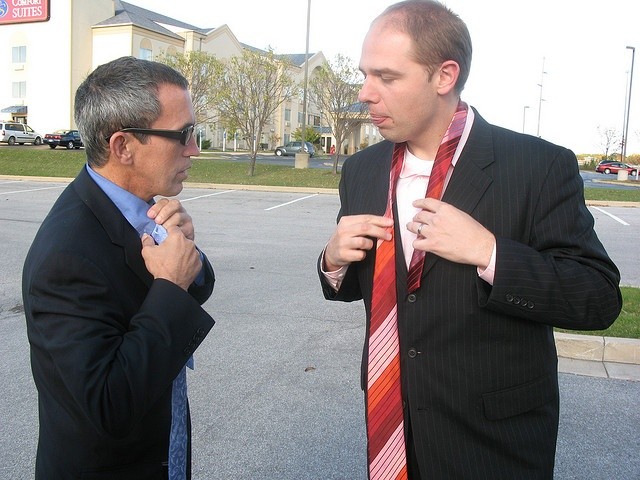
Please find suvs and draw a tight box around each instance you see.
[274,140,317,156]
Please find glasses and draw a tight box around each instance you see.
[117,126,196,145]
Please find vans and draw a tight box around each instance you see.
[0,122,43,146]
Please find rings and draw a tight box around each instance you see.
[417,222,424,234]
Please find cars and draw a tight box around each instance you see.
[44,129,85,149]
[595,160,640,176]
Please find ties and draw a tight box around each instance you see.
[149,221,193,479]
[366,101,468,480]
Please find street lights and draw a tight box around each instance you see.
[523,106,530,135]
[621,46,636,164]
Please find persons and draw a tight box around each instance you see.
[318,0,622,480]
[21,56,215,479]
